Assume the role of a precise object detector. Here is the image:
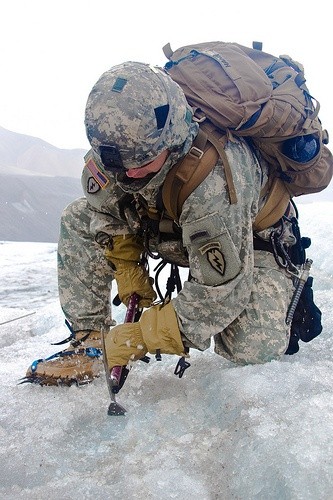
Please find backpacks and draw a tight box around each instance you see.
[164,41,333,234]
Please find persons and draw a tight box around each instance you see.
[25,61,312,386]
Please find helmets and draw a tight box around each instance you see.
[83,60,195,173]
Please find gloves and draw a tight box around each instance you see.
[105,300,191,396]
[100,234,157,309]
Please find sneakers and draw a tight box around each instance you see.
[24,330,112,385]
